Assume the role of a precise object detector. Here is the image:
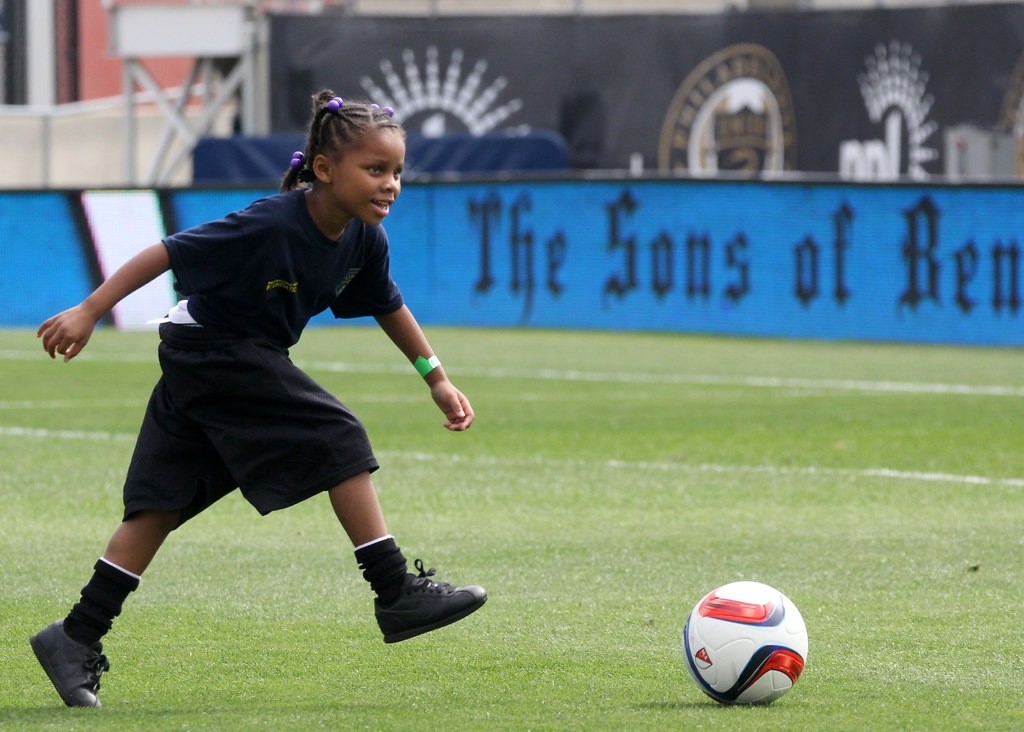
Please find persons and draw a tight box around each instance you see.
[28,90,490,712]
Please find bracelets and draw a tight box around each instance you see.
[415,355,439,376]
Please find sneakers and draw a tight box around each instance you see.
[372,559,486,644]
[29,618,109,707]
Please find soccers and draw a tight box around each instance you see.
[682,580,811,706]
